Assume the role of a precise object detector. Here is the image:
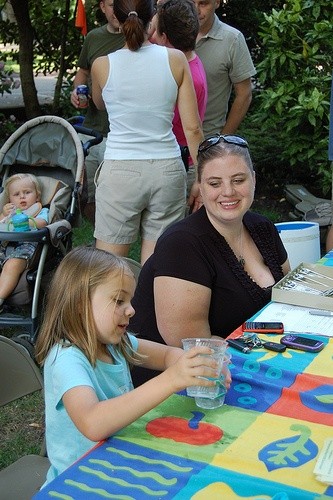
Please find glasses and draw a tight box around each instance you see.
[197,133,250,159]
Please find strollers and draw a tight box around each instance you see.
[0,114,104,364]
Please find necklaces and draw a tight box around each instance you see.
[226,241,246,267]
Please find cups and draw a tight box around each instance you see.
[181,339,229,400]
[194,351,232,410]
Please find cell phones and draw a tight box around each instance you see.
[242,322,283,334]
[280,335,323,352]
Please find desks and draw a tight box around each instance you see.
[29,247,333,500]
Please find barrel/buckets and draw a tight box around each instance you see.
[274,221,321,270]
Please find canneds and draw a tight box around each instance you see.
[76,85,89,108]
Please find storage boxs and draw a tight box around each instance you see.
[272,262,333,310]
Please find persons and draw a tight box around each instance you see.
[183,0,257,220]
[89,0,210,266]
[67,0,137,220]
[31,245,233,495]
[0,173,48,317]
[120,133,295,400]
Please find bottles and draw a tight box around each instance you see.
[7,208,37,232]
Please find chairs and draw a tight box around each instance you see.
[0,334,52,500]
